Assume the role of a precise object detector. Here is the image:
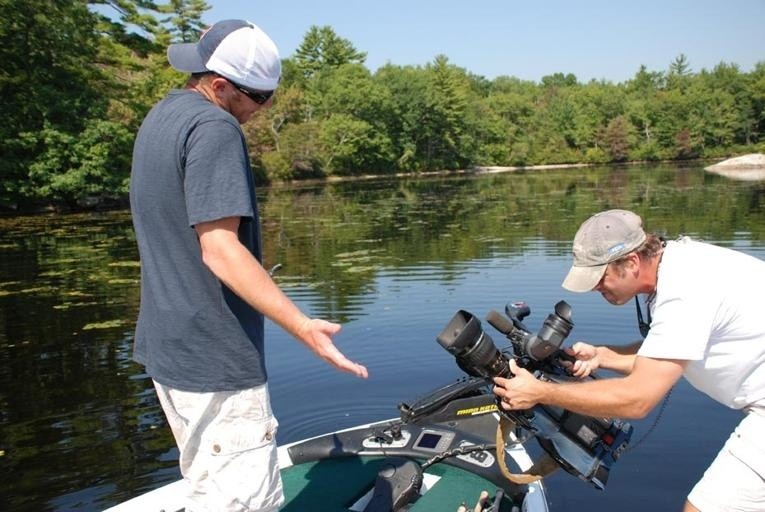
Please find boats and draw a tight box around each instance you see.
[99,404,548,512]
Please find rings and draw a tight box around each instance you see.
[503,397,507,402]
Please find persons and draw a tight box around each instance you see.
[129,18,369,512]
[486,207,765,511]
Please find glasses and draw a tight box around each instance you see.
[218,74,274,105]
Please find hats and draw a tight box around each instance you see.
[166,19,282,91]
[561,209,647,295]
